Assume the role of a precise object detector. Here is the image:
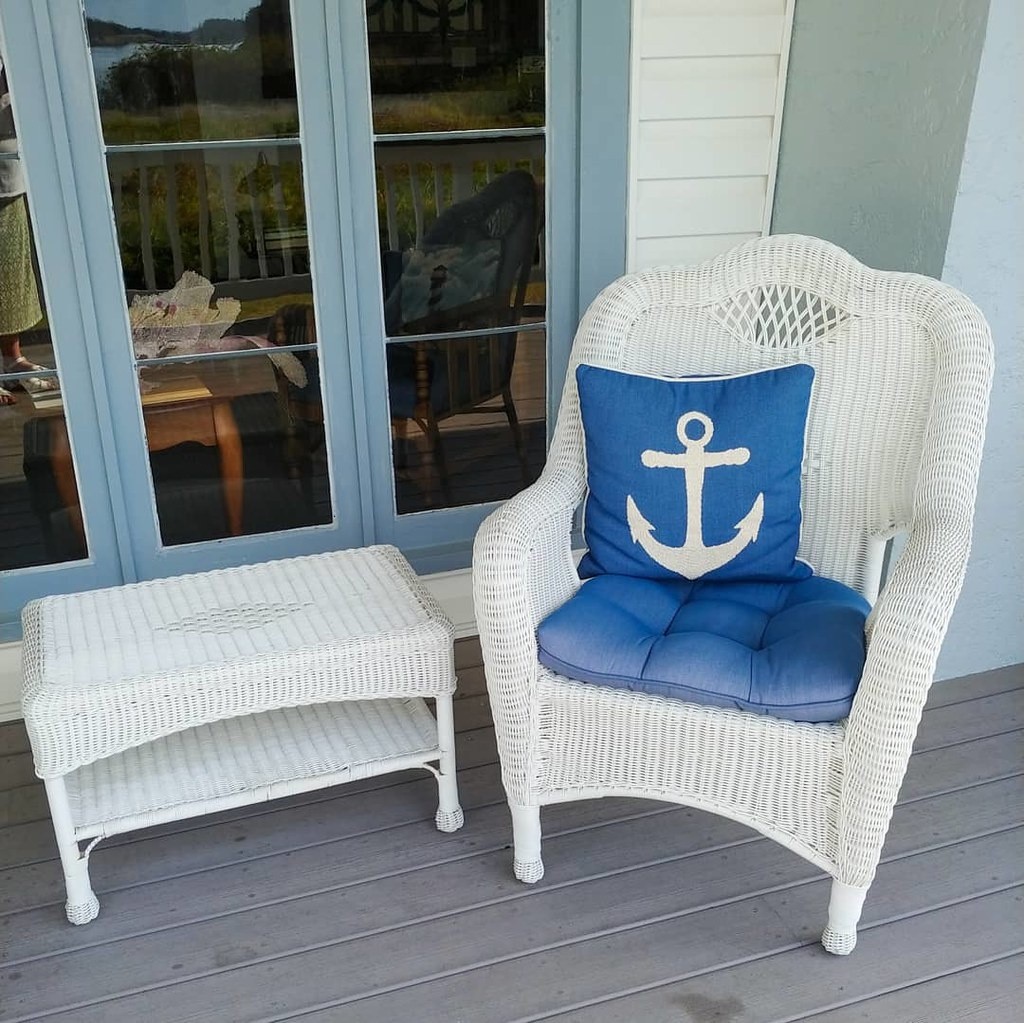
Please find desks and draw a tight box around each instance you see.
[21,544,463,926]
[17,343,274,549]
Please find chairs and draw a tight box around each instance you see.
[271,292,521,498]
[470,235,995,954]
[393,168,535,329]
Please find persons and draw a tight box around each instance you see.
[0,51,60,406]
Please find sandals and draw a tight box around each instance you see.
[0,387,17,406]
[3,357,48,372]
[3,378,56,394]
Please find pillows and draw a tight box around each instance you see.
[575,362,813,584]
